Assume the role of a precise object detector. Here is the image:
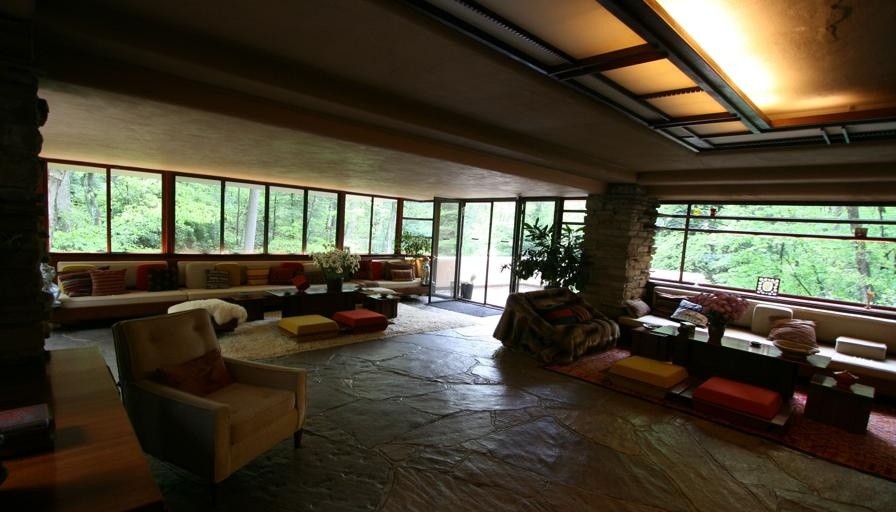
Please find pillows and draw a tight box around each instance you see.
[59,263,189,298]
[652,291,689,320]
[566,301,595,323]
[203,262,305,290]
[355,259,418,283]
[669,297,709,328]
[542,309,574,328]
[834,336,889,363]
[624,296,652,320]
[752,303,795,339]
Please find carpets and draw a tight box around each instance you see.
[537,346,896,485]
[423,296,504,319]
[211,302,475,362]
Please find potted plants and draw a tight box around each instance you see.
[290,270,312,293]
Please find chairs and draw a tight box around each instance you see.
[506,285,622,365]
[109,308,308,493]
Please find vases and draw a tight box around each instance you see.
[322,267,345,294]
[705,311,728,342]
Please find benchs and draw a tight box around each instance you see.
[346,258,437,303]
[54,259,188,335]
[620,282,896,414]
[173,260,327,322]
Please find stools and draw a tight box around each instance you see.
[169,299,249,337]
[608,353,690,390]
[694,375,785,421]
[277,313,341,345]
[333,310,390,335]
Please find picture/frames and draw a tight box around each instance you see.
[755,276,780,296]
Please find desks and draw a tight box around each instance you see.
[632,326,662,361]
[361,285,400,320]
[1,343,167,512]
[653,324,834,404]
[264,284,360,321]
[804,374,876,435]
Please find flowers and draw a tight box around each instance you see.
[307,244,364,276]
[686,289,750,328]
